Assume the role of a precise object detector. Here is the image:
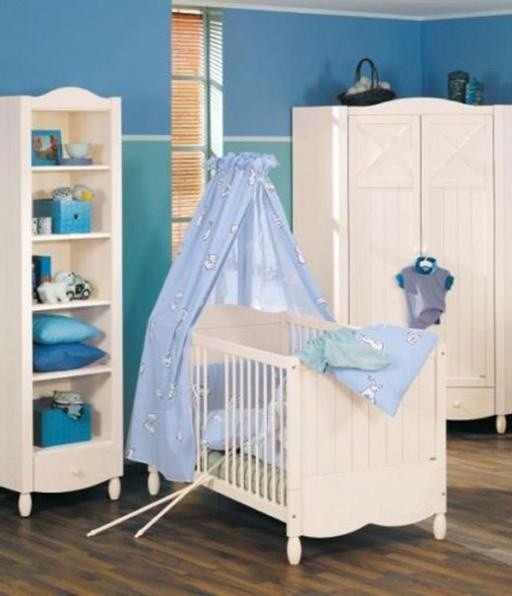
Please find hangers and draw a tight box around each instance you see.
[416,249,432,268]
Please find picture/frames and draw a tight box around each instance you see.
[30,128,62,165]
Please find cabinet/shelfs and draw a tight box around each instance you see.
[0,86,124,518]
[291,96,512,434]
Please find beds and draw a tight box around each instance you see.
[143,299,448,566]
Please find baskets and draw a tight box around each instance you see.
[337,57,396,106]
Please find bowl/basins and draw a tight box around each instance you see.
[63,142,91,158]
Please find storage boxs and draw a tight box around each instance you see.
[33,198,91,232]
[32,395,92,448]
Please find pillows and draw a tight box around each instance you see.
[32,344,107,371]
[32,313,103,344]
[201,406,280,450]
